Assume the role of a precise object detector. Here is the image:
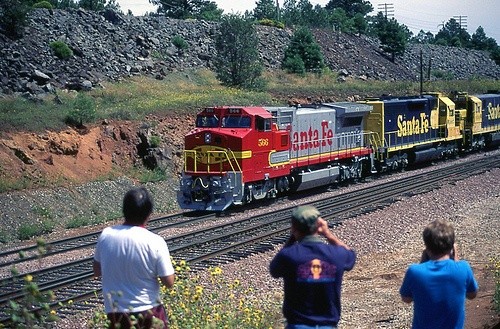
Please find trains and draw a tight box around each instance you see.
[176,91,500,212]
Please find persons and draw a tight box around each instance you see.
[268,205,356,329]
[92,185,174,329]
[398,217,479,329]
